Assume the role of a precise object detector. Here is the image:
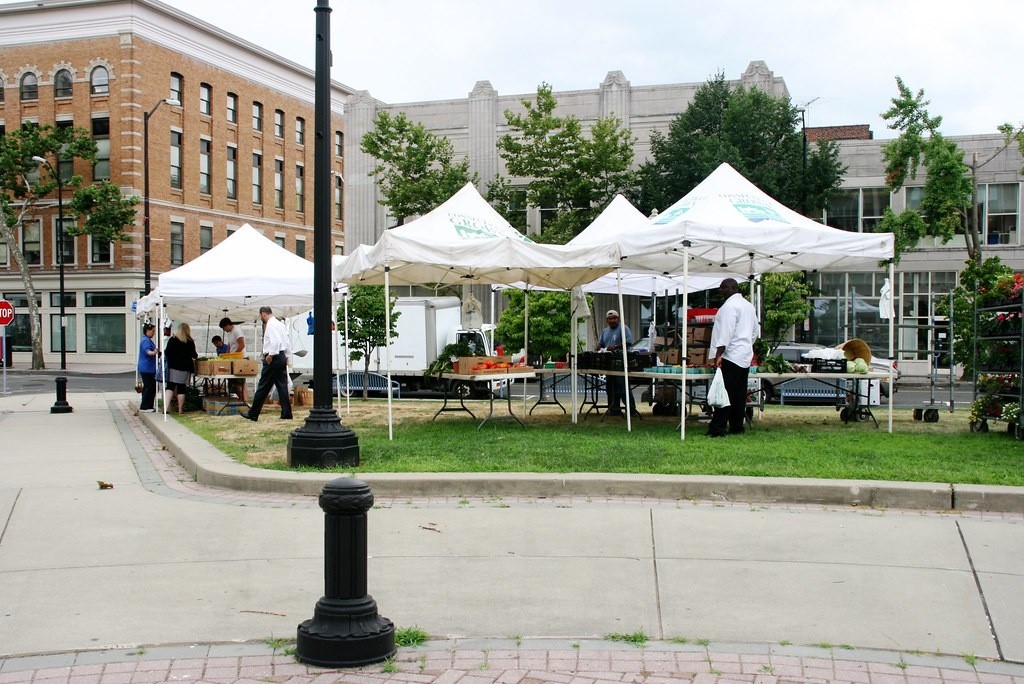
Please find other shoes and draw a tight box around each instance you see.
[240,411,258,421]
[140,408,155,413]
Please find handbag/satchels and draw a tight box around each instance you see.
[706,367,731,409]
[155,356,168,382]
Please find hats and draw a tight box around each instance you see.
[606,310,619,320]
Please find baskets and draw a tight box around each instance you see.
[800,356,847,373]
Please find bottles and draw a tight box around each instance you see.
[299,391,309,407]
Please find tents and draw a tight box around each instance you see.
[135,162,898,433]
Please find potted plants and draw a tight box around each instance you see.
[451,340,474,374]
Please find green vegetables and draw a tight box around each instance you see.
[753,338,794,374]
[424,342,473,388]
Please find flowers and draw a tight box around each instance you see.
[968,272,1023,424]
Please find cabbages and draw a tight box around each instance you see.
[843,348,868,373]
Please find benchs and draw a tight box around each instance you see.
[541,374,607,401]
[780,377,845,406]
[332,371,400,399]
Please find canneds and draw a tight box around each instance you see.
[496,345,503,356]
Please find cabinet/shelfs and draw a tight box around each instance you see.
[651,288,709,417]
[836,287,954,423]
[969,279,1024,441]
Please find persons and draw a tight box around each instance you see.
[219,317,249,403]
[137,323,161,413]
[162,323,198,417]
[703,277,759,437]
[212,335,238,401]
[239,307,293,422]
[593,310,637,420]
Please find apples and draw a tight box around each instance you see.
[471,360,512,370]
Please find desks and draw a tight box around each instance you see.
[188,374,252,416]
[748,372,898,428]
[529,369,753,430]
[422,371,535,432]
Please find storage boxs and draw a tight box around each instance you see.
[205,399,231,415]
[201,397,215,411]
[195,360,258,376]
[800,355,847,373]
[577,351,658,372]
[214,401,248,416]
[289,385,313,406]
[653,309,719,401]
[844,380,882,406]
[458,356,534,375]
